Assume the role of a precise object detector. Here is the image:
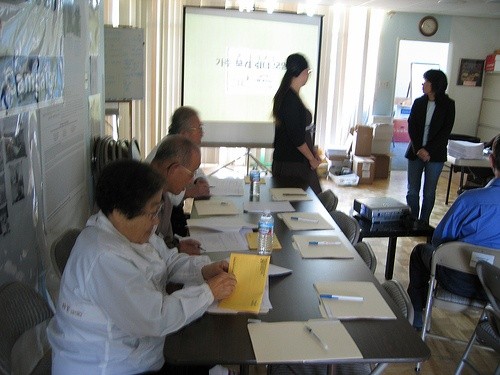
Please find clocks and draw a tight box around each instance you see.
[420,16,439,36]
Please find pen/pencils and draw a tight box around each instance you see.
[320,295,364,302]
[283,193,307,196]
[309,242,341,245]
[291,216,318,223]
[304,322,327,349]
[174,234,206,252]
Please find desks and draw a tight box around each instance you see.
[161,177,432,375]
[446,153,491,205]
[353,215,435,280]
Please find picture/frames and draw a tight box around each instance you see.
[456,58,485,87]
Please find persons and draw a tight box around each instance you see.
[271,53,322,195]
[147,134,201,256]
[50,159,237,375]
[403,70,456,222]
[405,133,500,352]
[142,107,209,199]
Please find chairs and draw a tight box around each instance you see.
[265,280,414,375]
[0,283,52,375]
[353,241,377,274]
[449,134,482,194]
[330,209,361,246]
[414,241,500,375]
[49,226,83,278]
[90,135,143,188]
[317,189,338,213]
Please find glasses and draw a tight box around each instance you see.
[146,202,163,220]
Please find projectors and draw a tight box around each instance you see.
[354,197,412,223]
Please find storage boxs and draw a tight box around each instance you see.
[485,51,500,71]
[313,104,411,186]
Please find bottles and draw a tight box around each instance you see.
[257,209,274,256]
[250,166,261,196]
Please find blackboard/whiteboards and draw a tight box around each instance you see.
[104,24,145,100]
